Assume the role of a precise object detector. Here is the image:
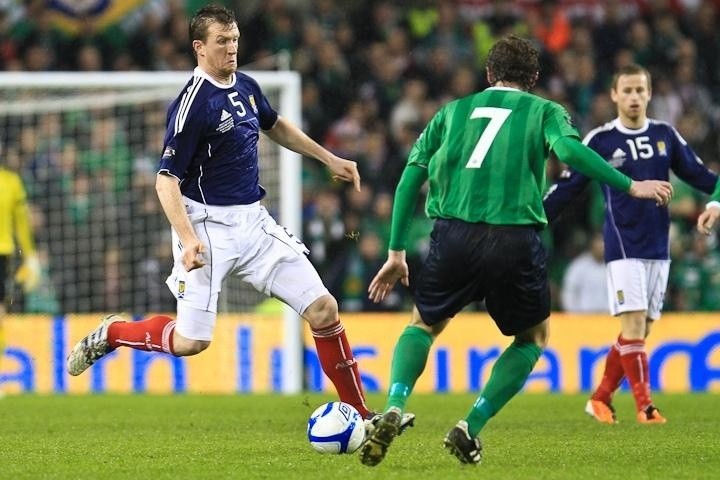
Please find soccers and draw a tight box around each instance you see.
[309,403,365,454]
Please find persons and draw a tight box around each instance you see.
[0,0,720,315]
[542,65,719,424]
[64,6,414,437]
[359,35,673,466]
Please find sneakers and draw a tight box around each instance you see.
[359,407,415,468]
[585,397,618,424]
[443,419,483,466]
[636,404,668,424]
[66,313,126,377]
